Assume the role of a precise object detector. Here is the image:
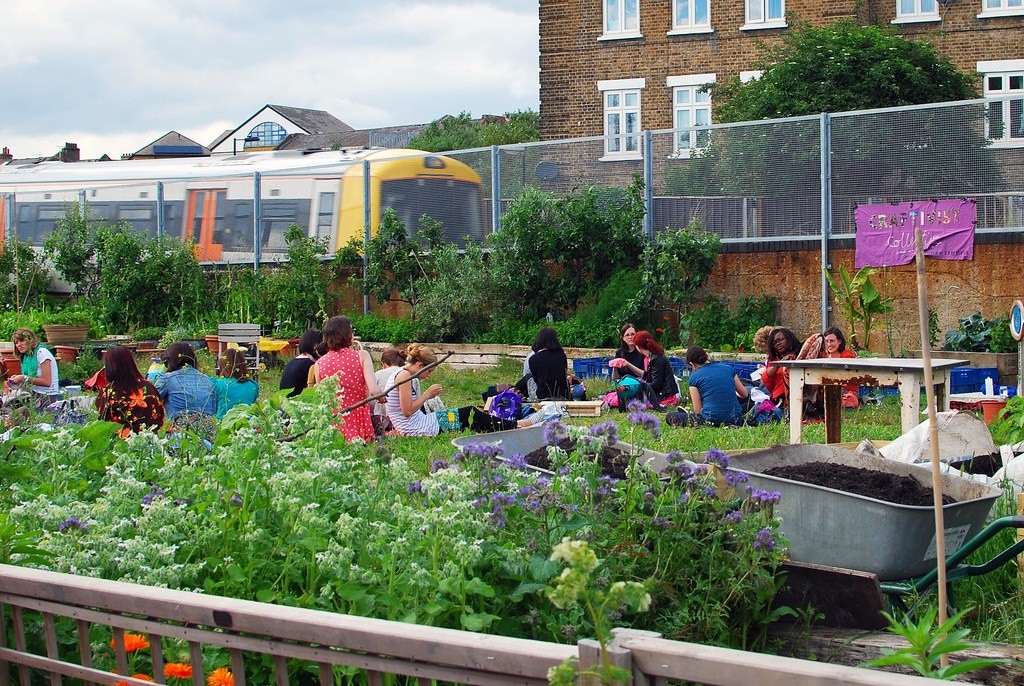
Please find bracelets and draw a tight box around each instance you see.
[25,375,28,381]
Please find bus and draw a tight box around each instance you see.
[0,146,489,294]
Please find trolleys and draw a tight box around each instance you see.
[453,424,743,519]
[712,444,1024,626]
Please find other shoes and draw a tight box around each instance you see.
[677,406,689,413]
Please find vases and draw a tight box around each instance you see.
[983,400,1006,427]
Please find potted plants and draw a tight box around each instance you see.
[274,320,306,356]
[0,312,226,384]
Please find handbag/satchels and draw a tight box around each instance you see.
[490,390,523,420]
[603,389,620,408]
[53,399,88,425]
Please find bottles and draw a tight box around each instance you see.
[685,407,691,427]
[985,376,1008,399]
[597,392,606,412]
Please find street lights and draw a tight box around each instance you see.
[505,148,526,189]
[233,137,260,155]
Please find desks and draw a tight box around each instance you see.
[770,358,973,445]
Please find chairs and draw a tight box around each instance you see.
[215,323,261,375]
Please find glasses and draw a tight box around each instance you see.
[164,361,169,368]
[350,327,357,334]
[623,333,637,338]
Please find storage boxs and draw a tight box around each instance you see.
[949,366,1017,414]
[859,386,899,398]
[575,355,760,383]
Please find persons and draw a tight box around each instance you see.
[665,346,749,430]
[611,323,679,408]
[210,348,259,420]
[736,326,858,416]
[11,328,58,410]
[523,327,571,401]
[153,342,217,444]
[95,347,164,435]
[279,315,420,443]
[384,342,545,436]
